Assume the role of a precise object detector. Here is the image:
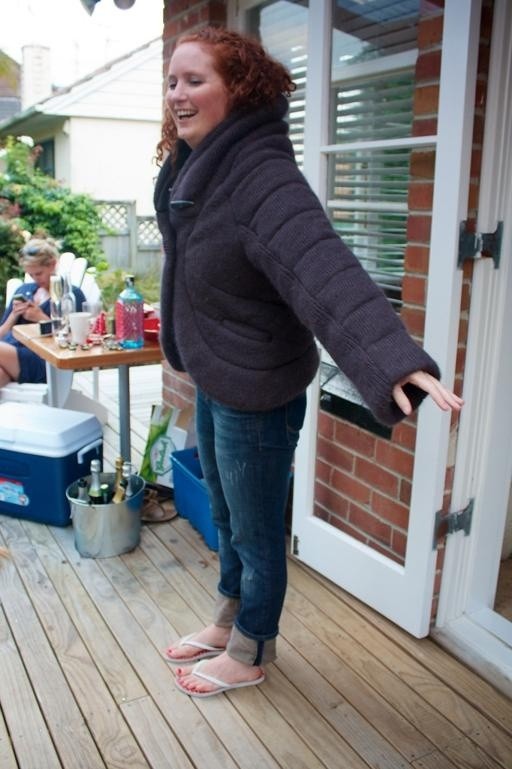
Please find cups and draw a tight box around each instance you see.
[68,311,96,344]
[82,301,105,327]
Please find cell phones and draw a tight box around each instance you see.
[13,294,27,303]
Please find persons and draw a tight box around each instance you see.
[0,237,85,392]
[152,25,465,700]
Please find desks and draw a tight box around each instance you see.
[11,317,167,473]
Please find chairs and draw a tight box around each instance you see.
[5,252,102,408]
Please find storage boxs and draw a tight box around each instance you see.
[169,444,294,552]
[0,401,104,529]
[140,402,196,490]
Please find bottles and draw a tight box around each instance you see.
[75,455,139,509]
[49,274,78,345]
[115,276,145,349]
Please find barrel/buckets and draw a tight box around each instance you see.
[65,471,146,559]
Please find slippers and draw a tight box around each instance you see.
[140,489,177,522]
[165,633,265,697]
